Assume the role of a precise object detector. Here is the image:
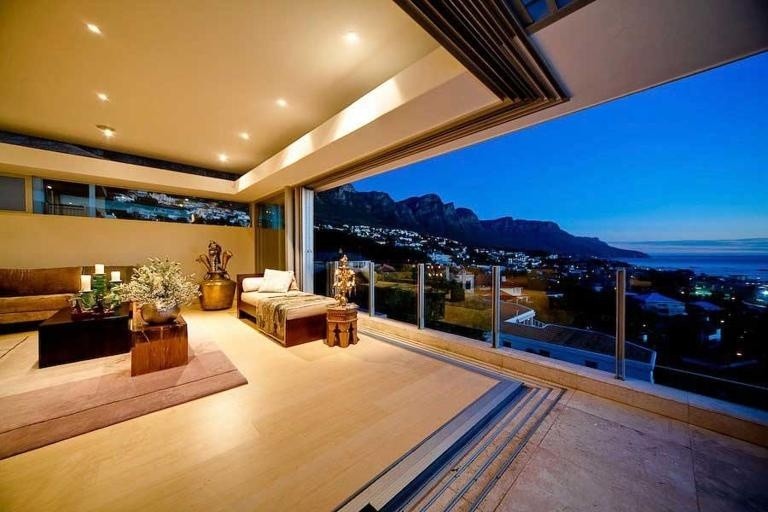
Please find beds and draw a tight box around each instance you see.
[236,271,336,348]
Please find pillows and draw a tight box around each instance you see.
[241,277,263,293]
[256,269,293,293]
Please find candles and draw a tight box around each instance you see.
[110,271,120,282]
[79,274,90,291]
[94,265,105,274]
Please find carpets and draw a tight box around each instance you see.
[1,333,249,462]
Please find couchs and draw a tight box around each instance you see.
[0,267,133,325]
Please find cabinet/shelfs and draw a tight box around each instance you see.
[128,314,188,378]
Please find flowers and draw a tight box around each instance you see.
[110,256,202,313]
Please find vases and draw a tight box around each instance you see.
[140,303,178,322]
